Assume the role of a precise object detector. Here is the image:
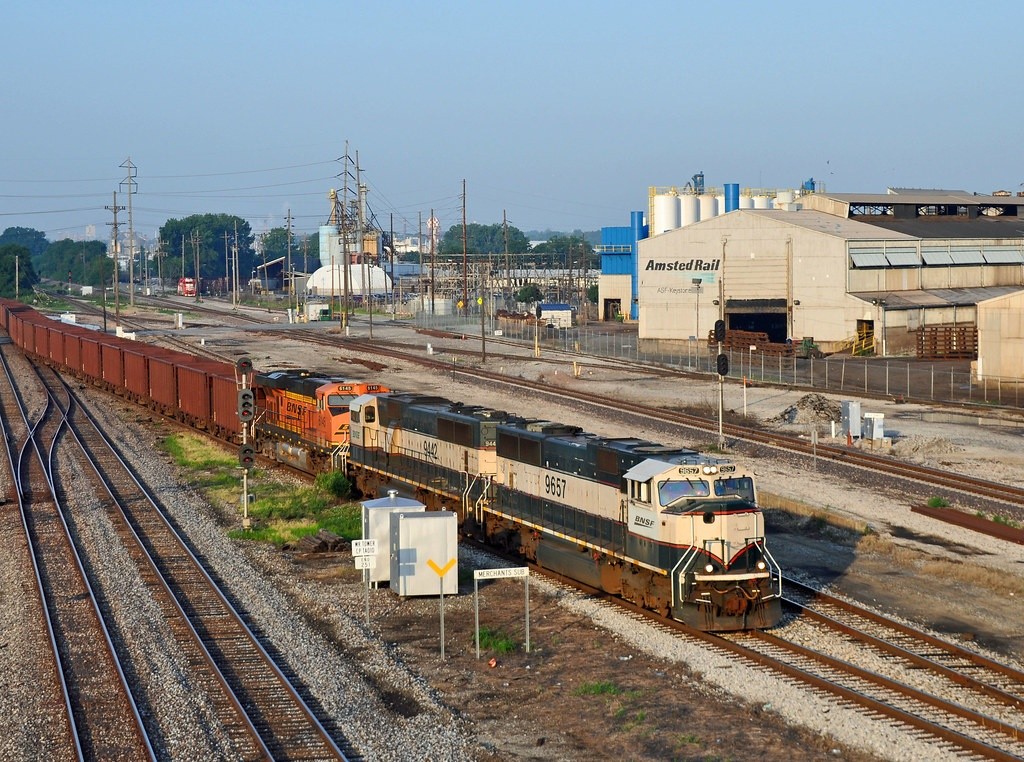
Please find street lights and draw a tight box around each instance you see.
[692,277,703,372]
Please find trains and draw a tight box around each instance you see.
[0,298,784,629]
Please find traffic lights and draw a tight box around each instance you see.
[536,306,542,319]
[236,358,252,374]
[237,389,254,421]
[717,354,729,376]
[714,320,726,341]
[239,444,254,468]
